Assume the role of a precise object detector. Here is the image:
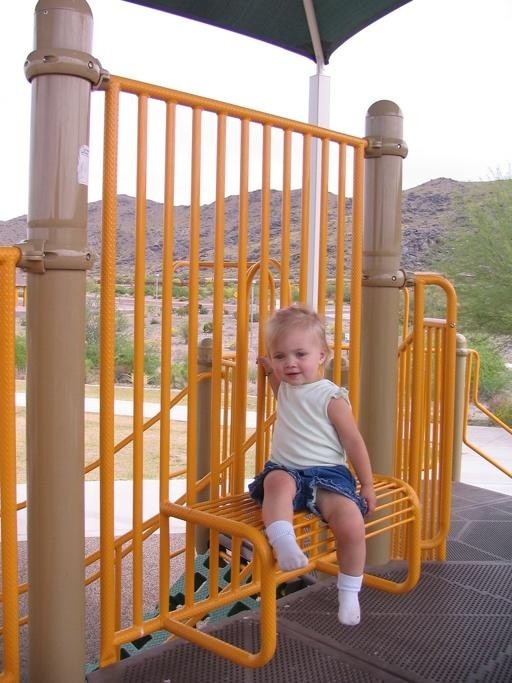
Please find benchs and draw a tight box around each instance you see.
[158,470,421,667]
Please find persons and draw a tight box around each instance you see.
[248,303,378,626]
